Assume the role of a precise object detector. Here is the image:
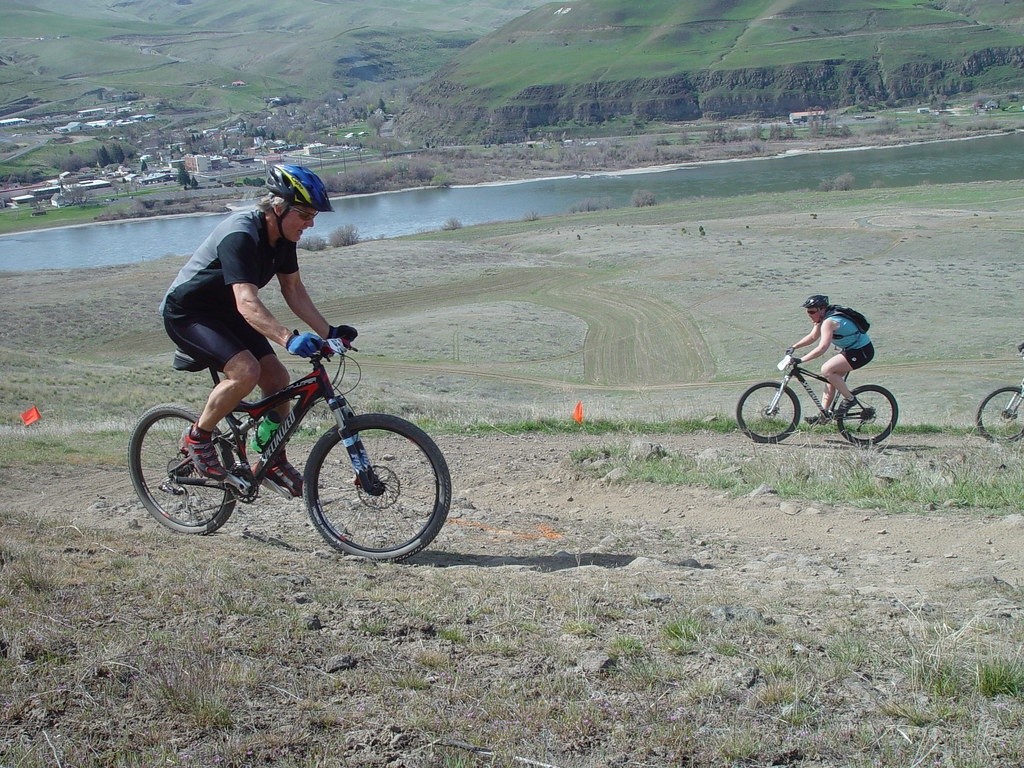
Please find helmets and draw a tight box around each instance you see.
[800,294,829,308]
[264,164,334,212]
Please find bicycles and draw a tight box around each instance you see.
[127,332,452,561]
[735,351,899,444]
[975,349,1024,444]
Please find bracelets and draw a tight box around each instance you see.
[284,332,292,348]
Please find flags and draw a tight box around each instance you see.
[572,401,583,423]
[19,406,42,426]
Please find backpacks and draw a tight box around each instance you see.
[823,302,870,350]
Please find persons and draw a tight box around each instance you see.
[785,295,875,426]
[159,164,359,497]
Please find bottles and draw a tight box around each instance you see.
[250,410,282,453]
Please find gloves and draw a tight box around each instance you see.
[789,357,801,365]
[783,346,795,356]
[325,325,358,351]
[285,329,324,359]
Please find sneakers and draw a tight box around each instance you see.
[252,452,304,497]
[804,416,830,425]
[177,423,228,482]
[837,396,858,415]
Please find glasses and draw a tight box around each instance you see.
[288,206,320,220]
[806,310,818,314]
